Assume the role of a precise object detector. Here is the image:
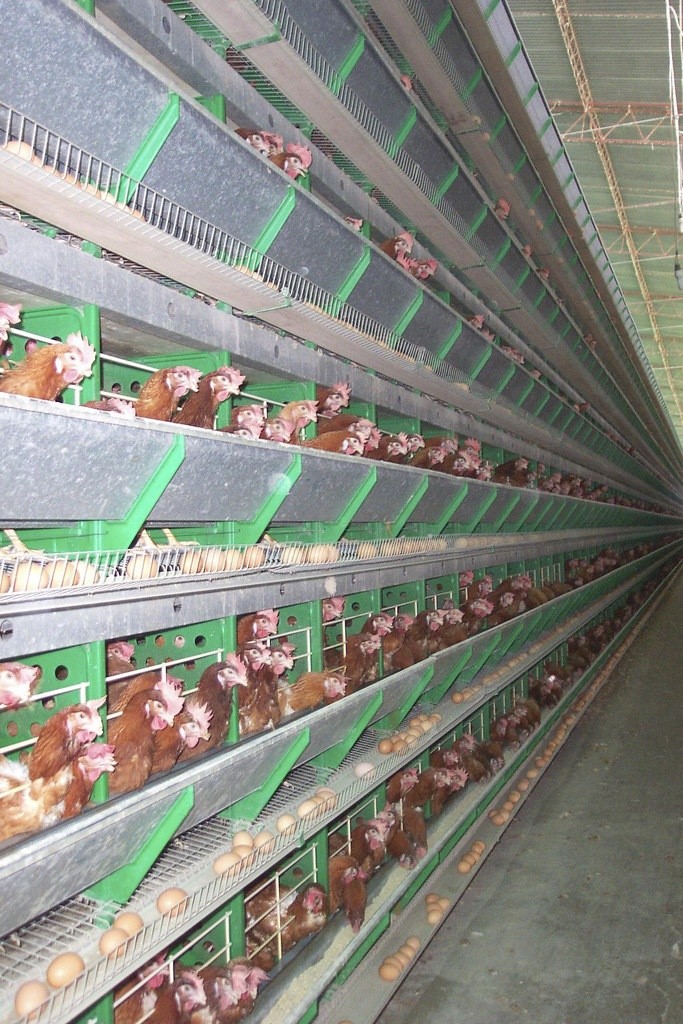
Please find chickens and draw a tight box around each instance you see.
[0,73,683,1024]
